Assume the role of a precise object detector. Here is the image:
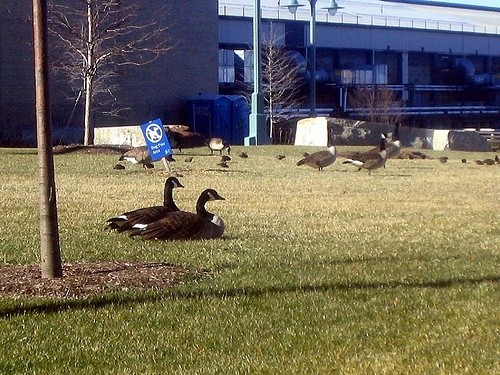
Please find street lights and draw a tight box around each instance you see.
[277,0,346,117]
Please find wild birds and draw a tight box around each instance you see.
[276,155,286,161]
[337,138,387,176]
[112,143,194,171]
[204,137,248,167]
[296,139,337,172]
[380,133,500,167]
[103,176,227,241]
